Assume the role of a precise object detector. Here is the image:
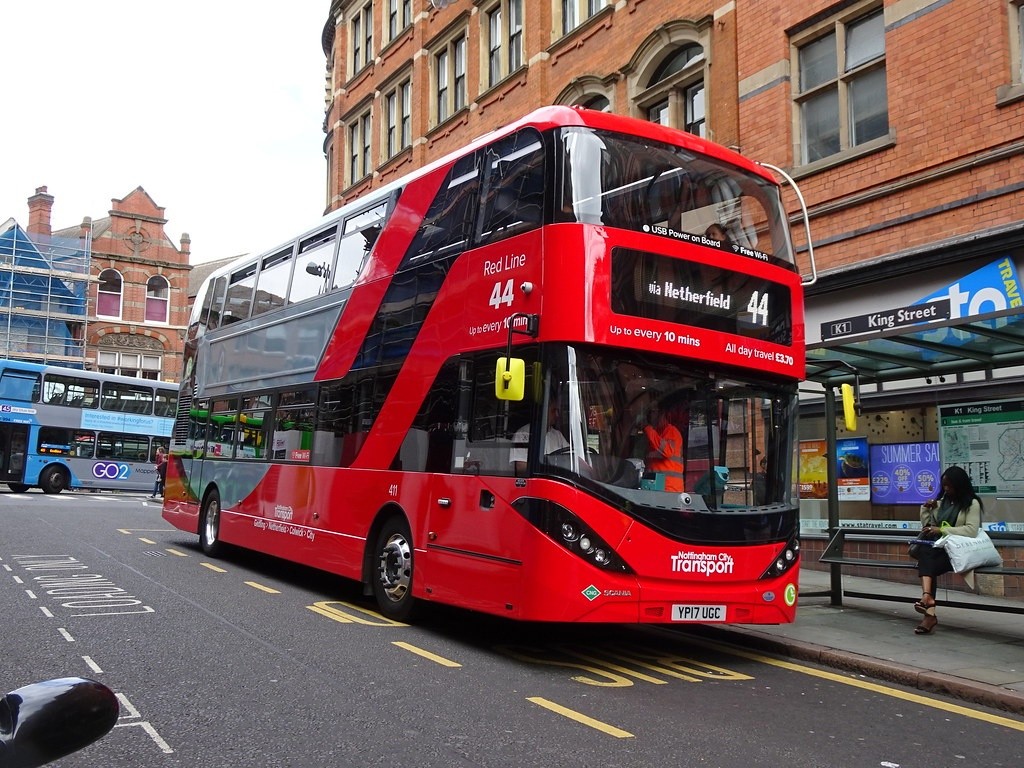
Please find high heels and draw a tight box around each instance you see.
[914,616,938,635]
[914,591,936,616]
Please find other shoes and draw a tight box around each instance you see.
[147,495,155,500]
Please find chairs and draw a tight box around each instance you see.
[69,396,87,406]
[90,398,175,417]
[49,393,66,404]
[32,390,40,402]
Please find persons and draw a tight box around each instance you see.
[509,403,574,478]
[636,405,684,493]
[914,467,985,636]
[146,447,168,498]
[749,456,767,507]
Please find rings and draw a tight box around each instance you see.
[928,527,931,531]
[931,499,934,501]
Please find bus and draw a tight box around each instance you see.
[0,358,180,494]
[160,103,864,627]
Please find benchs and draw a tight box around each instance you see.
[818,528,1024,576]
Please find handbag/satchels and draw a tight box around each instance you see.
[940,521,1003,574]
[907,509,939,560]
